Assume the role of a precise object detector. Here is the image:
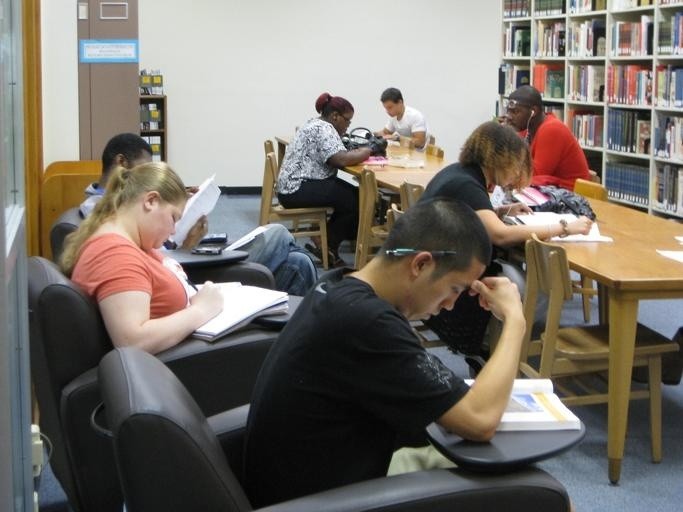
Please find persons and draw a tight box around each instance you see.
[58,161,223,356]
[373,87,426,154]
[78,131,318,296]
[277,93,387,266]
[238,196,528,511]
[415,121,592,366]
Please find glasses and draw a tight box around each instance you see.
[503,98,532,108]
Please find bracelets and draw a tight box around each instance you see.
[393,134,400,142]
[559,220,571,236]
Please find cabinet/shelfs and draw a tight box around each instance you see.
[497,1,683,221]
[138,93,167,166]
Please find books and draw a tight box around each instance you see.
[191,281,288,341]
[139,68,163,161]
[363,157,426,169]
[502,205,610,243]
[461,378,582,434]
[497,0,681,216]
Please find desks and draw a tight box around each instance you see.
[374,172,683,486]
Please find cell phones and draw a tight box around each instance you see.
[191,246,222,255]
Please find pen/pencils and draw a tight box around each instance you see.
[186,280,198,291]
[385,248,457,257]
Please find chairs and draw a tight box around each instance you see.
[517,232,679,461]
[48,206,278,291]
[561,178,607,324]
[568,165,609,302]
[25,253,307,509]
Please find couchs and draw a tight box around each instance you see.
[97,343,574,508]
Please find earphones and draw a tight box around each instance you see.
[530,110,535,117]
